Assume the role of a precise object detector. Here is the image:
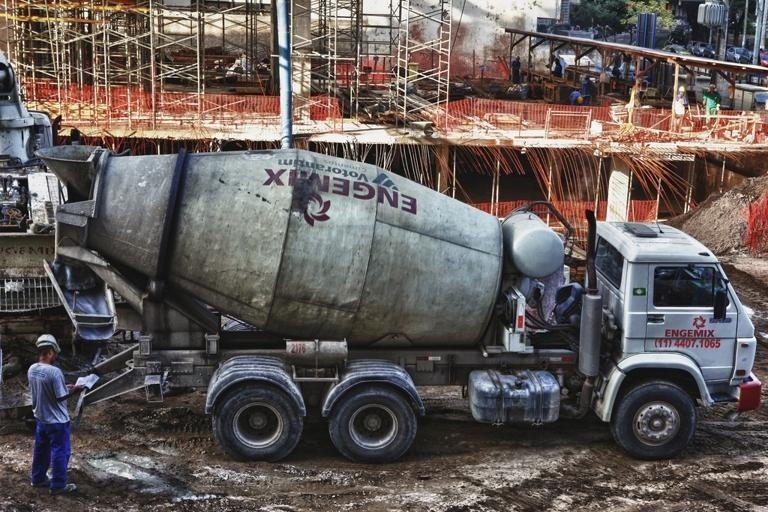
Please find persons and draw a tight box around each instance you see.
[509,54,722,116]
[27,333,89,496]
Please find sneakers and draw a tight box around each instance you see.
[30,476,53,487]
[48,483,77,495]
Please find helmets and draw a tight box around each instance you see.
[35,334,61,353]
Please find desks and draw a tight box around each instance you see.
[523,65,672,105]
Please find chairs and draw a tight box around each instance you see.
[657,271,693,306]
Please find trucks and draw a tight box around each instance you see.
[31,142,763,466]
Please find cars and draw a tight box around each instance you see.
[750,51,768,67]
[725,47,750,64]
[663,40,717,60]
